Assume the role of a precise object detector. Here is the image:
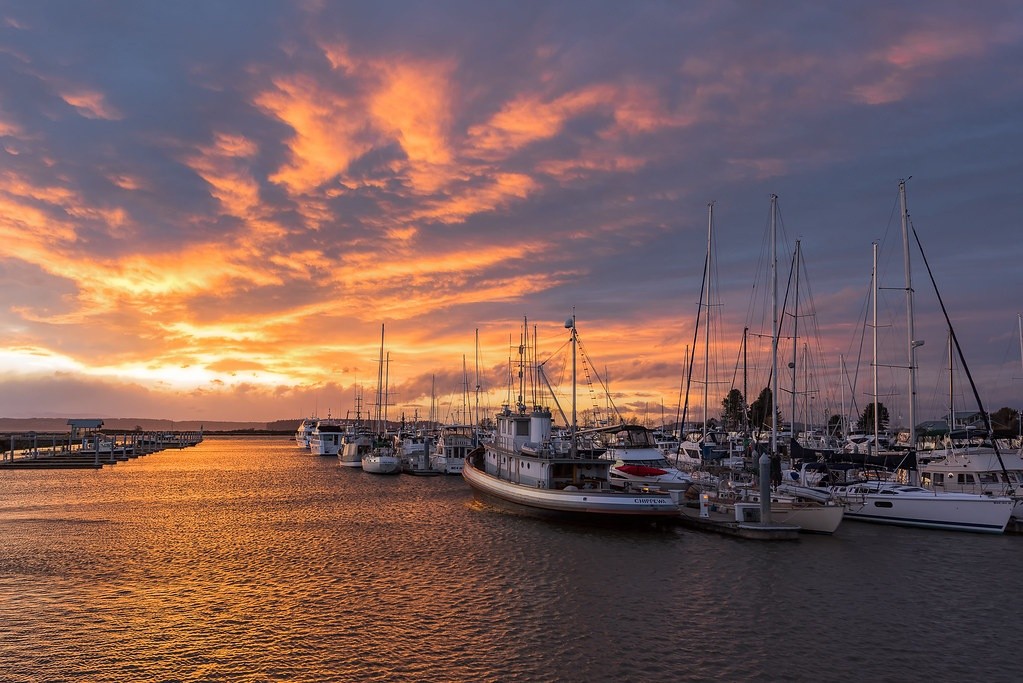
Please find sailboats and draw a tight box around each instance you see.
[334,172,1023,538]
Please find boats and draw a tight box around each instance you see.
[294,416,320,449]
[308,413,344,456]
[920,427,1023,524]
[137,434,203,444]
[81,438,133,454]
[576,424,694,496]
